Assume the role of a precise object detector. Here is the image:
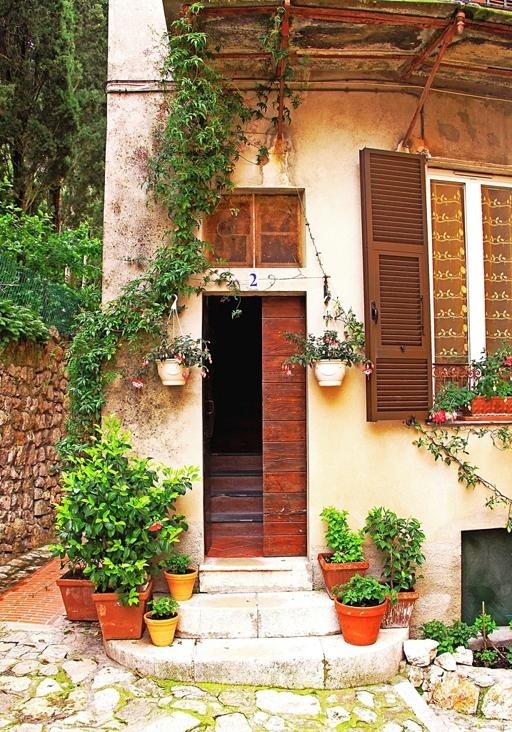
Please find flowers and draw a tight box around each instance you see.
[276,298,375,381]
[128,335,214,389]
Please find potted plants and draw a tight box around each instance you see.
[466,341,511,417]
[317,503,429,646]
[45,408,200,648]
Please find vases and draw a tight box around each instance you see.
[313,358,348,388]
[153,358,190,386]
